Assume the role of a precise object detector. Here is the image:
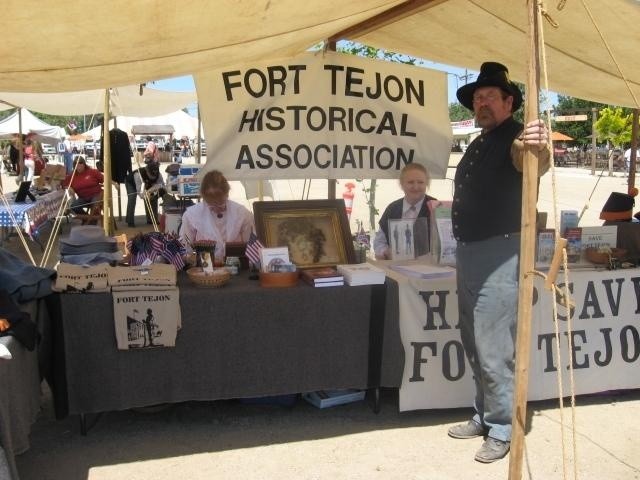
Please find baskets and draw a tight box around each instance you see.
[187,267,231,288]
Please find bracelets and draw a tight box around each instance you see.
[539,142,549,153]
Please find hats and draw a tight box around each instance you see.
[59,226,117,253]
[456,61,522,112]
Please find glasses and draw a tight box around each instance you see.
[473,91,504,102]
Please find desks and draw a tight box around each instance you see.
[0,282,53,463]
[60,265,399,437]
[388,257,640,419]
[1,186,66,254]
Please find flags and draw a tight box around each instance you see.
[125,230,187,272]
[245,233,265,263]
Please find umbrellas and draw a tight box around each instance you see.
[552,131,574,141]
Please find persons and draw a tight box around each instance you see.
[177,169,257,262]
[392,225,400,254]
[404,223,413,255]
[124,163,167,228]
[374,162,440,261]
[143,136,161,167]
[8,132,43,187]
[445,59,553,467]
[60,155,104,225]
[142,307,155,347]
[623,147,640,185]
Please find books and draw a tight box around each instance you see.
[257,245,296,273]
[336,261,386,287]
[301,267,344,283]
[299,276,344,288]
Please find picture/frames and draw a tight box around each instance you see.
[252,198,360,271]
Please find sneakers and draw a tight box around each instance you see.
[156,221,160,224]
[148,221,153,225]
[90,220,97,225]
[128,224,134,228]
[82,213,89,225]
[474,437,510,463]
[449,419,488,438]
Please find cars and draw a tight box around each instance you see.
[40,137,207,157]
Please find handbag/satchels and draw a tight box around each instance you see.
[144,155,154,163]
